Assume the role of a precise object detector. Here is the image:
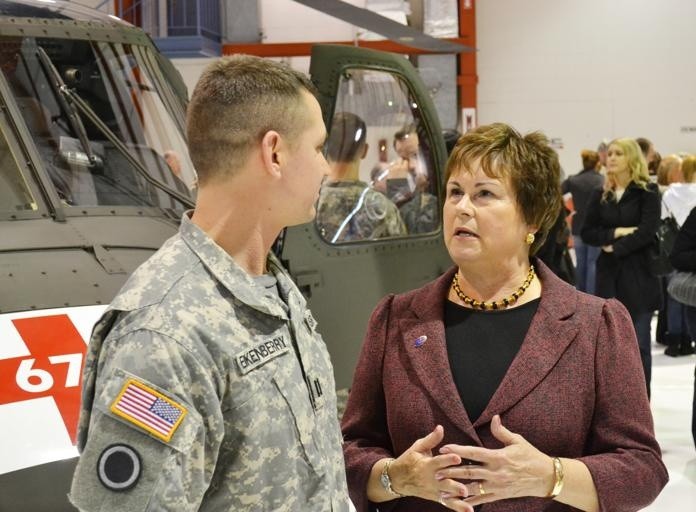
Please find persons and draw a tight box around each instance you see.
[390,129,463,234]
[581,136,662,402]
[315,111,409,242]
[635,137,696,357]
[66,51,355,512]
[371,121,419,199]
[337,122,671,511]
[596,141,608,176]
[561,149,605,293]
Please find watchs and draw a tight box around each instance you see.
[377,454,405,503]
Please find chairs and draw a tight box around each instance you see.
[0,97,98,214]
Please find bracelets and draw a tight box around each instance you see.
[546,451,568,503]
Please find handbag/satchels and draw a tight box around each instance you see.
[666,271,696,307]
[642,218,679,276]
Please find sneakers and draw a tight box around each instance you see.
[665,341,694,356]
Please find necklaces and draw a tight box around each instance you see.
[450,264,537,314]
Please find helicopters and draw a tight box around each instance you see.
[0,0,481,509]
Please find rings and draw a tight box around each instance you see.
[476,481,488,496]
